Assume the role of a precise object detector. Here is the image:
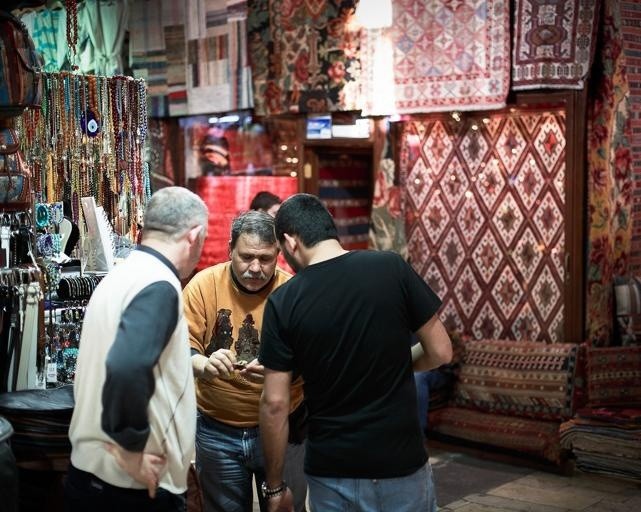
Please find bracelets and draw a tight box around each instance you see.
[35,233,105,378]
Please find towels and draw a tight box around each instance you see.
[361,1,600,118]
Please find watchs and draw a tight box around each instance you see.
[260,481,287,499]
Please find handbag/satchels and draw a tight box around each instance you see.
[0,9,44,109]
[0,127,34,212]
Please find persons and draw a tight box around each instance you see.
[413,364,459,442]
[250,192,283,218]
[258,194,454,512]
[183,209,307,512]
[68,185,209,512]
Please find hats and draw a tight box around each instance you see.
[243,315,254,324]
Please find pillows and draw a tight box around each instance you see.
[615,284,641,345]
[581,342,641,406]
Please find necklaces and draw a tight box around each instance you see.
[66,0,80,70]
[10,70,152,247]
